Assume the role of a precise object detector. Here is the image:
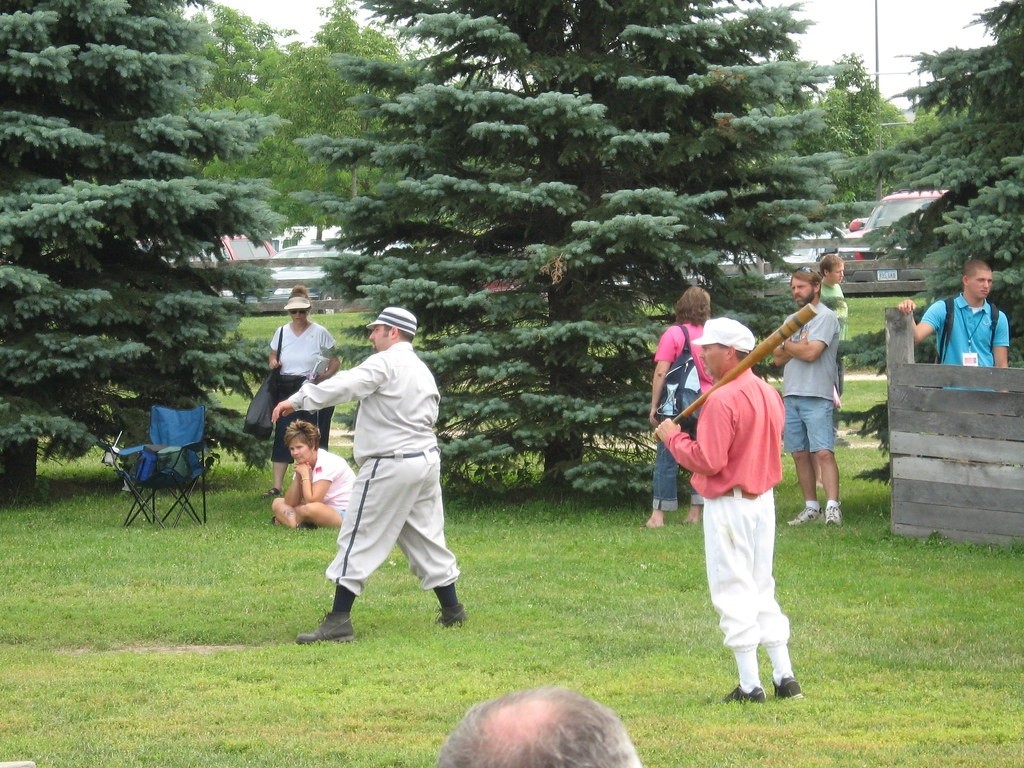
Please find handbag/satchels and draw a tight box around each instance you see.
[243,366,282,441]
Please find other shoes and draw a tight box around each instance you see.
[272,516,318,530]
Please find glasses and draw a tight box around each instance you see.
[291,310,308,314]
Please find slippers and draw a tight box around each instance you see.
[262,488,283,497]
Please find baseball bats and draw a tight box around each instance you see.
[652,303,818,443]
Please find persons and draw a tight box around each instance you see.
[897,258,1009,393]
[271,419,357,530]
[773,254,847,528]
[273,307,469,644]
[655,317,804,705]
[434,687,644,768]
[262,285,340,499]
[637,286,711,531]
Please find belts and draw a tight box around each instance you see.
[722,487,758,499]
[371,446,437,458]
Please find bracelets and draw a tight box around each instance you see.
[292,473,300,482]
[779,340,785,351]
[302,478,311,481]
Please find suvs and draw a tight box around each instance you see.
[839,188,949,257]
[192,235,275,296]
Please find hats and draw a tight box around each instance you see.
[284,296,311,309]
[692,316,755,354]
[366,306,417,336]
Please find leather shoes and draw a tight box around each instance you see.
[436,603,468,629]
[296,612,355,644]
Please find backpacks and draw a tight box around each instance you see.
[655,323,702,427]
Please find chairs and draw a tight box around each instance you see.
[96,405,207,528]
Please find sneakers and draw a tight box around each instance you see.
[722,683,767,703]
[772,676,804,700]
[825,505,842,526]
[786,506,824,526]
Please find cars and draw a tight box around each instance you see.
[717,227,842,283]
[250,245,357,313]
